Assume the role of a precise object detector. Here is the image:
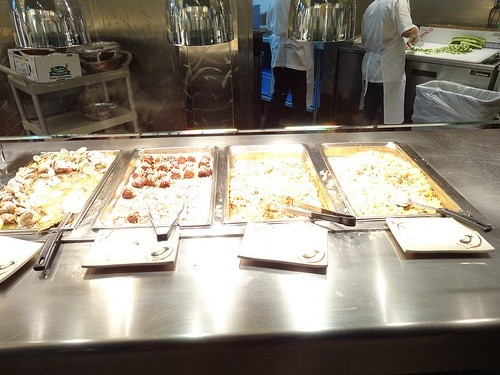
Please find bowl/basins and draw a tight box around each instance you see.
[80,57,121,74]
[80,41,119,61]
[80,102,118,121]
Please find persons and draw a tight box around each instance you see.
[258,2,314,128]
[359,0,419,125]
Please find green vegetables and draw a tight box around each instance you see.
[410,36,485,55]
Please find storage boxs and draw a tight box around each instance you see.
[262,68,323,107]
[7,49,82,84]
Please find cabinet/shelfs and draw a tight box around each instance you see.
[261,36,352,126]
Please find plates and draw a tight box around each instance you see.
[0,235,44,285]
[237,222,329,269]
[81,228,179,269]
[385,217,495,253]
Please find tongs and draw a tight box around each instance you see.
[148,200,184,239]
[272,197,356,227]
